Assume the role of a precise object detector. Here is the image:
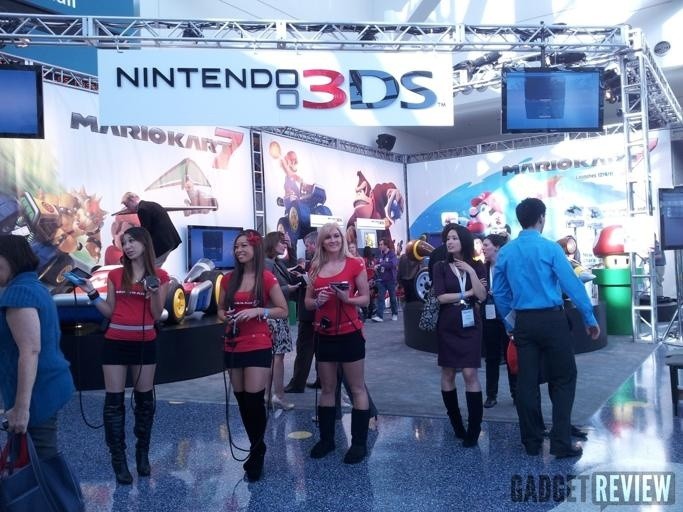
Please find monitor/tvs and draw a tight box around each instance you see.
[187,225,243,270]
[658,187,683,251]
[501,66,606,135]
[0,64,45,140]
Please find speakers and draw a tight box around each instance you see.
[376,134,396,151]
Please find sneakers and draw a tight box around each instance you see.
[371,313,398,323]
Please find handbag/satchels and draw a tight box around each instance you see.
[505,333,518,375]
[418,291,439,329]
[0,427,86,512]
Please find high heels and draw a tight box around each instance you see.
[263,394,297,412]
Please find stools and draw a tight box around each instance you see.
[664,352,682,416]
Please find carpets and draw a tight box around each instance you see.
[72,306,662,426]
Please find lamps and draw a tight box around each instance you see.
[538,50,588,68]
[454,50,501,83]
[597,67,662,129]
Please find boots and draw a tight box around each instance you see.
[440,388,467,439]
[132,388,155,477]
[310,405,336,459]
[103,390,134,485]
[233,388,268,480]
[343,407,368,464]
[462,390,483,448]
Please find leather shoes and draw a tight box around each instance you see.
[283,381,322,393]
[525,426,588,458]
[484,397,498,409]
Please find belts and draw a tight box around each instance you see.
[516,304,562,313]
